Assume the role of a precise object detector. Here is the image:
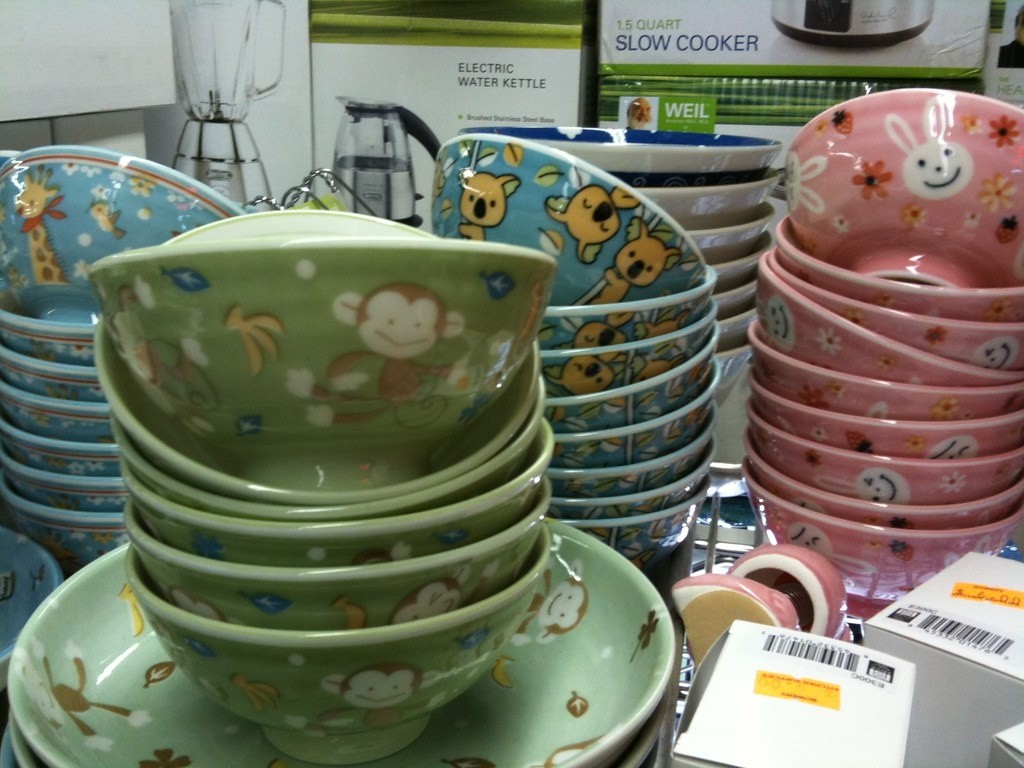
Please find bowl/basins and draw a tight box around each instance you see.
[90,88,1024,768]
[0,144,246,693]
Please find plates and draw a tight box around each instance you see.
[7,514,675,768]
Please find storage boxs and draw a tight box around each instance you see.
[313,0,1024,234]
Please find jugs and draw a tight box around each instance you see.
[328,95,444,229]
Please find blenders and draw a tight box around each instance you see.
[165,0,288,213]
